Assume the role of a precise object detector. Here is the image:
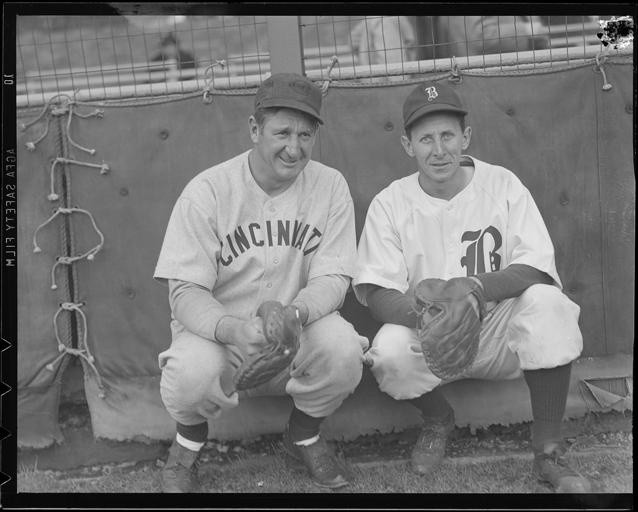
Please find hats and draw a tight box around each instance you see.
[401,81,468,131]
[253,72,325,126]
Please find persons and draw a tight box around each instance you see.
[152,37,197,72]
[153,73,369,494]
[351,83,592,493]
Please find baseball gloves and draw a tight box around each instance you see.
[234,302,303,389]
[412,279,485,377]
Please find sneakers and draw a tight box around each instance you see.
[410,405,456,476]
[158,437,203,493]
[280,421,352,489]
[531,446,591,493]
